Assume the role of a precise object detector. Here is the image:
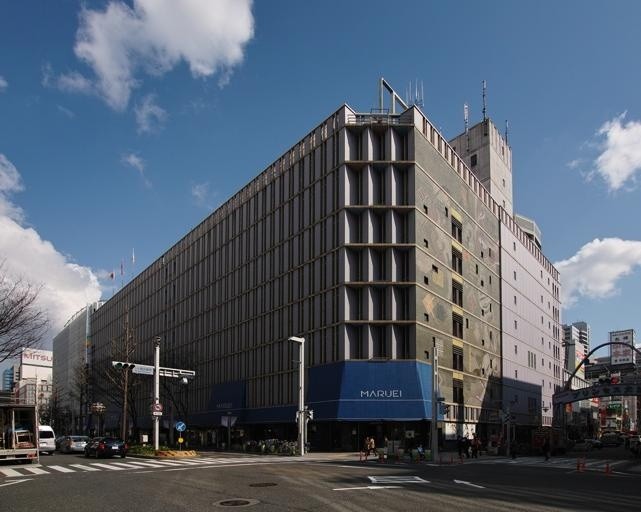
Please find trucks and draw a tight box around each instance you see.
[0,397,40,465]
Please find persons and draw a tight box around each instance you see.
[3,420,13,449]
[364,434,378,457]
[362,435,371,456]
[470,436,482,458]
[416,442,426,461]
[462,436,471,459]
[511,439,517,459]
[541,436,551,460]
[457,434,464,459]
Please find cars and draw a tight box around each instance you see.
[567,430,641,451]
[55,435,67,447]
[60,434,90,454]
[84,436,129,459]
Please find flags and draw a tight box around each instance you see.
[130,248,136,263]
[119,261,124,276]
[107,271,114,282]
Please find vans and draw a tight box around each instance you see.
[38,425,57,453]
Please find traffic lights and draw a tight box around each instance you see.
[440,402,451,415]
[115,362,135,370]
[306,409,315,420]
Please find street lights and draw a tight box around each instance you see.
[288,334,309,455]
